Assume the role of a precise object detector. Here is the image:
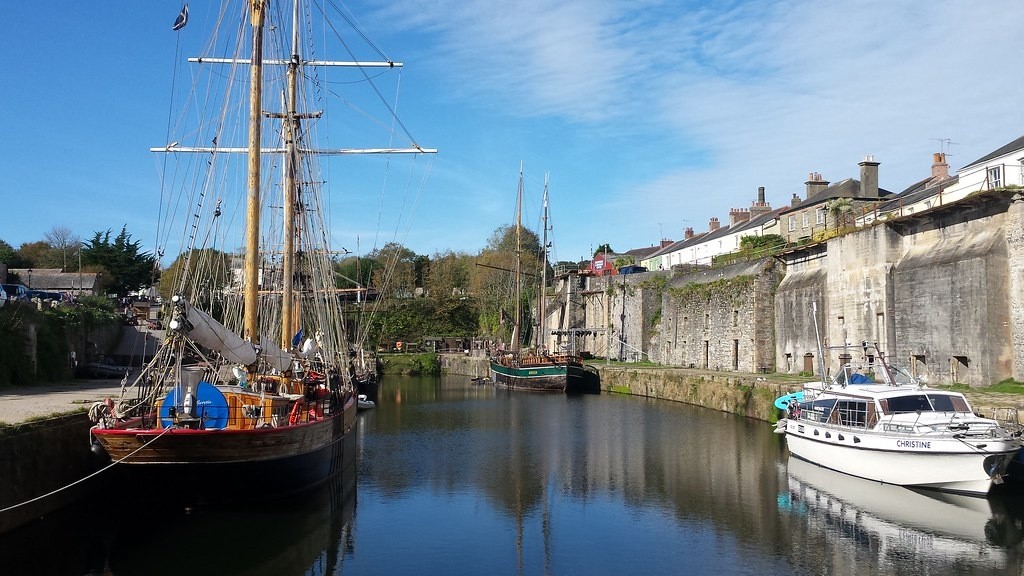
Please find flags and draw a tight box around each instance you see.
[173,3,189,31]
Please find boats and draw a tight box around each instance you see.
[771,302,1024,496]
[774,452,1024,570]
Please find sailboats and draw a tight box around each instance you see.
[473,160,601,395]
[87,0,438,500]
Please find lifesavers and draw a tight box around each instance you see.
[288,398,305,426]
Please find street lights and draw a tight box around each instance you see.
[27,267,32,288]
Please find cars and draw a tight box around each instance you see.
[147,320,163,330]
[0,282,78,309]
